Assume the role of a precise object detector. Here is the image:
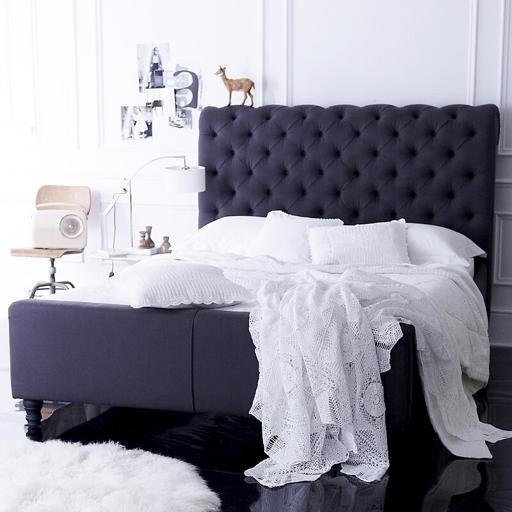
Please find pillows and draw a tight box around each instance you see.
[174,213,266,261]
[109,254,255,309]
[255,210,346,264]
[306,216,413,270]
[406,222,488,269]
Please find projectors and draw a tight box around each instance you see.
[32,209,87,250]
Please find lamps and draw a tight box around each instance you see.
[104,155,208,259]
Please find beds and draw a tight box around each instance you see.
[7,104,500,477]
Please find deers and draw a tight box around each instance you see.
[213,64,257,108]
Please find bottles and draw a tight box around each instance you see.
[139,226,170,252]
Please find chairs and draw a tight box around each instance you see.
[11,184,93,299]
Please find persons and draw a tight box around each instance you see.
[147,46,162,89]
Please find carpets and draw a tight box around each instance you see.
[5,434,221,512]
